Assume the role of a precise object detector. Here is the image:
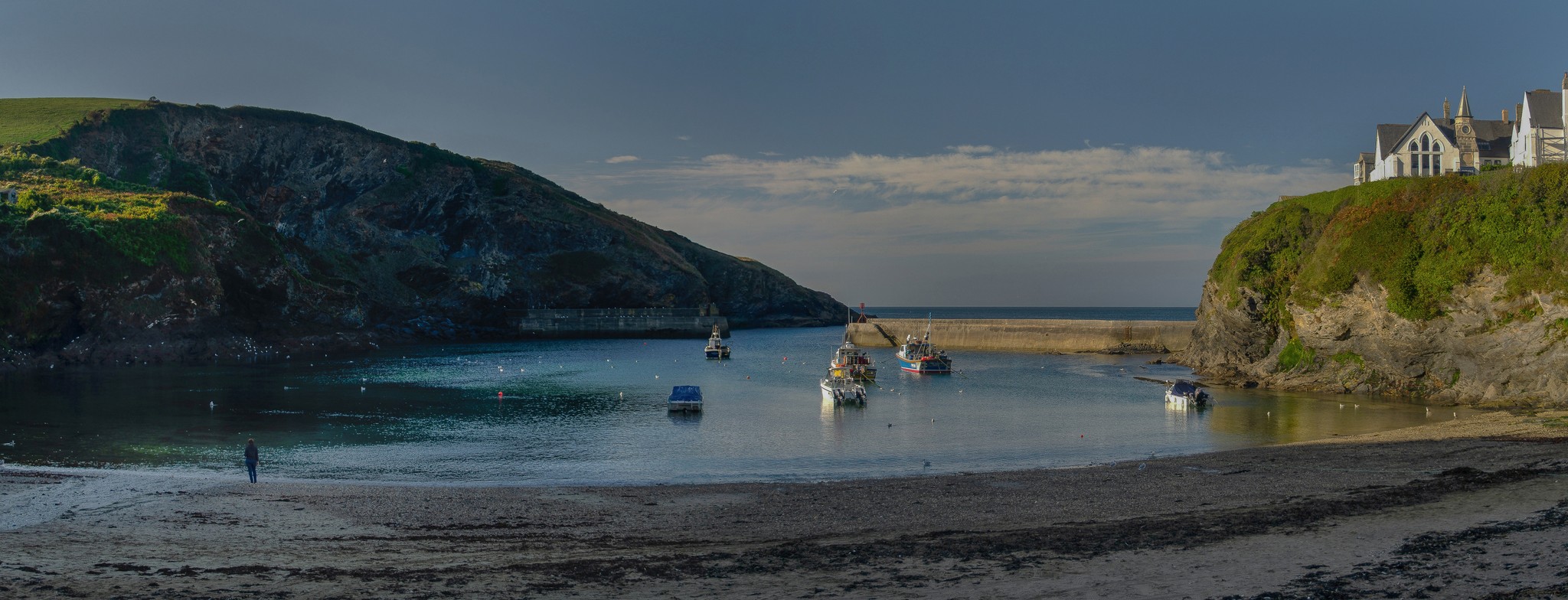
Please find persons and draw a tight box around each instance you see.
[244,439,259,484]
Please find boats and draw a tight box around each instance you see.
[820,366,868,404]
[667,384,705,412]
[831,332,877,382]
[1164,377,1211,405]
[704,319,732,359]
[895,312,952,374]
